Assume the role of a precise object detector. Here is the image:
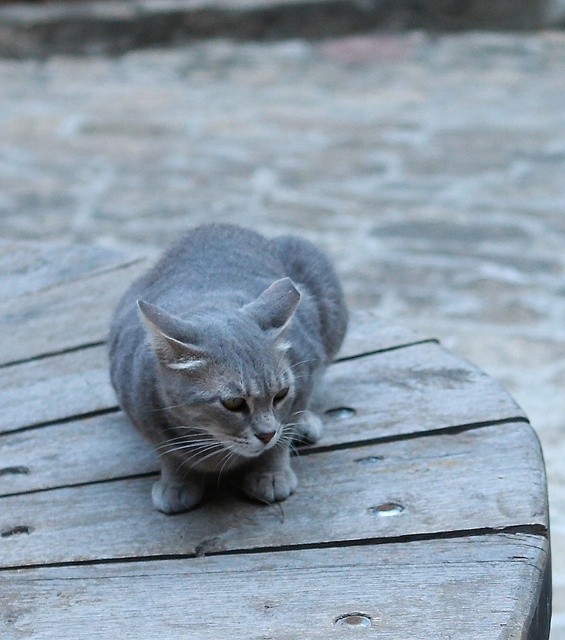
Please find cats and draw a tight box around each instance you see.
[104,218,351,514]
